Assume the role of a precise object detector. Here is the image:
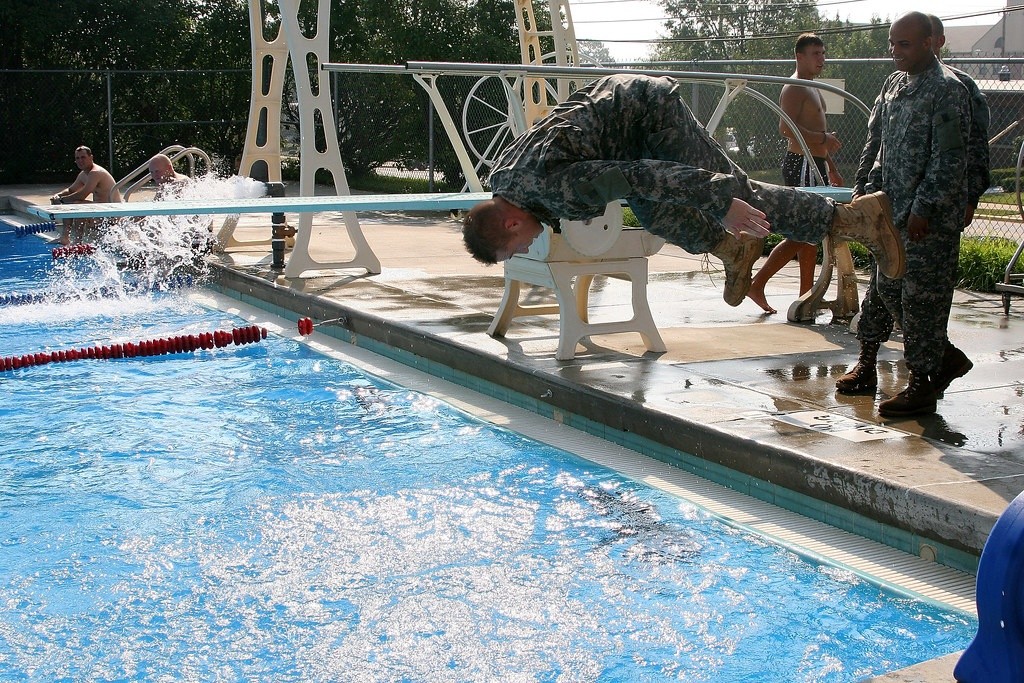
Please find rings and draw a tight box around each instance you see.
[913,233,919,236]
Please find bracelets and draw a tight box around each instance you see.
[820,130,826,145]
[828,169,839,173]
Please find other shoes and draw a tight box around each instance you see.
[830,191,905,280]
[836,364,878,390]
[711,230,769,306]
[936,347,972,391]
[879,372,936,417]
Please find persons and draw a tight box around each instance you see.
[462,73,905,307]
[836,14,993,398]
[865,11,975,417]
[743,33,841,314]
[129,154,212,231]
[45,145,124,247]
